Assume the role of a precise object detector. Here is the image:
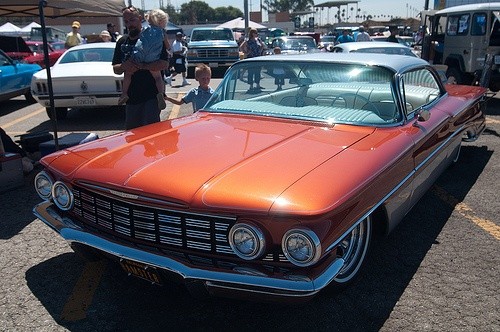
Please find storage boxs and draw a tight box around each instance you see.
[0,152,24,192]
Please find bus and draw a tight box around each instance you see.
[415,2,500,94]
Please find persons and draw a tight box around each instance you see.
[66,6,290,132]
[332,24,430,46]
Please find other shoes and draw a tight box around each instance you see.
[183,82,191,85]
[246,88,261,94]
[157,93,166,110]
[277,87,282,91]
[260,87,266,89]
[117,96,129,105]
[166,77,172,87]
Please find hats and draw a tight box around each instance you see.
[176,32,182,36]
[72,21,81,29]
[387,26,400,30]
[101,30,109,37]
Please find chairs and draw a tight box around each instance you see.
[86,53,99,60]
[315,94,346,108]
[279,96,318,107]
[378,99,413,114]
[362,102,397,117]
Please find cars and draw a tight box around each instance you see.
[0,25,240,122]
[267,23,422,58]
[31,50,490,301]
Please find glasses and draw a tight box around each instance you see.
[121,6,141,15]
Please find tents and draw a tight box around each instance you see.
[137,17,183,46]
[215,17,268,41]
[20,21,43,36]
[0,0,128,153]
[0,22,22,53]
